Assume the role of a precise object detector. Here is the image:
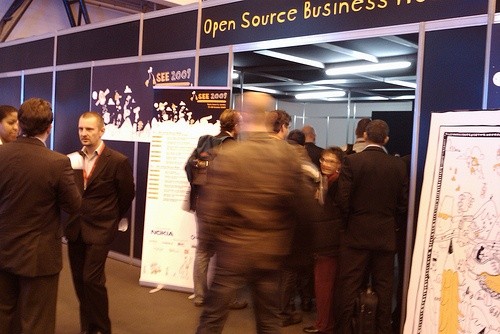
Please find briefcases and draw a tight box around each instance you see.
[353,290,377,334]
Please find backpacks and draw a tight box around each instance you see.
[184,136,234,185]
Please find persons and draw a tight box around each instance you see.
[185,92,413,334]
[0,98,84,334]
[0,105,20,145]
[334,118,407,334]
[61,112,136,334]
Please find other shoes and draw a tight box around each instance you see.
[229,299,249,309]
[193,295,204,305]
[284,299,296,311]
[303,326,323,334]
[301,297,312,311]
[281,314,302,327]
